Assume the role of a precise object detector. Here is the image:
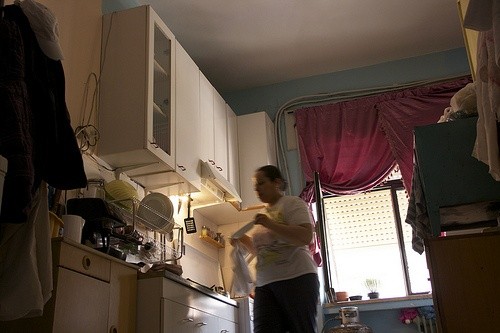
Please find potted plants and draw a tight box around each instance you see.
[364,277,382,299]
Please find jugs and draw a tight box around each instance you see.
[62,215,85,244]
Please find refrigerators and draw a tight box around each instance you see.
[411,116,500,333]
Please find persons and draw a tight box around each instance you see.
[227,162,321,333]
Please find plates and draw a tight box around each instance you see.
[138,195,175,233]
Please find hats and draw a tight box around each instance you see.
[19,0,65,61]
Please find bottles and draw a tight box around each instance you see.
[202,225,223,245]
[84,179,106,199]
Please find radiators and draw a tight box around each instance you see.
[404,314,437,333]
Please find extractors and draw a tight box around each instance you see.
[169,162,242,208]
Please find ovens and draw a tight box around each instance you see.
[239,297,255,333]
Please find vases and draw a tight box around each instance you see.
[336,292,349,301]
[349,295,363,301]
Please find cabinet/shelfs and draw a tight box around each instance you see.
[237,295,256,332]
[107,255,141,333]
[237,111,285,219]
[104,189,184,265]
[193,70,241,225]
[130,38,200,191]
[424,231,500,333]
[135,270,221,333]
[97,4,176,176]
[218,292,240,333]
[32,237,111,333]
